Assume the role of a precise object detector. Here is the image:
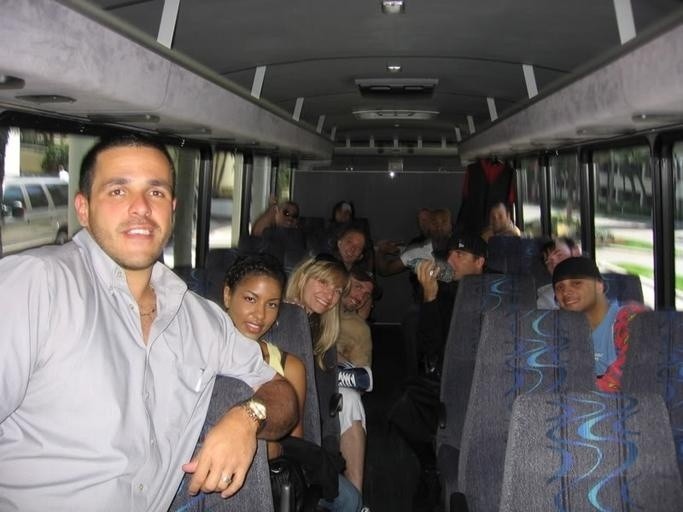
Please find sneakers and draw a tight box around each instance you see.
[336,360,373,392]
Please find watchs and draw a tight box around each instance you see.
[232,394,269,431]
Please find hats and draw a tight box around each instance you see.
[552,256,609,293]
[446,231,488,257]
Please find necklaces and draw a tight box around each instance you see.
[131,287,157,317]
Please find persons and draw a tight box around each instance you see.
[56,164,69,182]
[0,132,298,512]
[291,253,372,505]
[218,249,324,509]
[256,197,593,369]
[551,256,653,392]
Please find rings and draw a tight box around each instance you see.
[221,473,231,484]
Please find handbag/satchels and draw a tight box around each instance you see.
[267,456,322,512]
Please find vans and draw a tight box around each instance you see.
[2,175,68,255]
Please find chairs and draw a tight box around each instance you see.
[167,375,298,510]
[485,236,555,274]
[498,392,681,511]
[619,310,681,477]
[431,273,541,478]
[256,304,323,450]
[206,218,374,272]
[597,271,645,307]
[436,308,597,510]
[171,269,290,302]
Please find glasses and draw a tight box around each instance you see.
[282,209,299,219]
